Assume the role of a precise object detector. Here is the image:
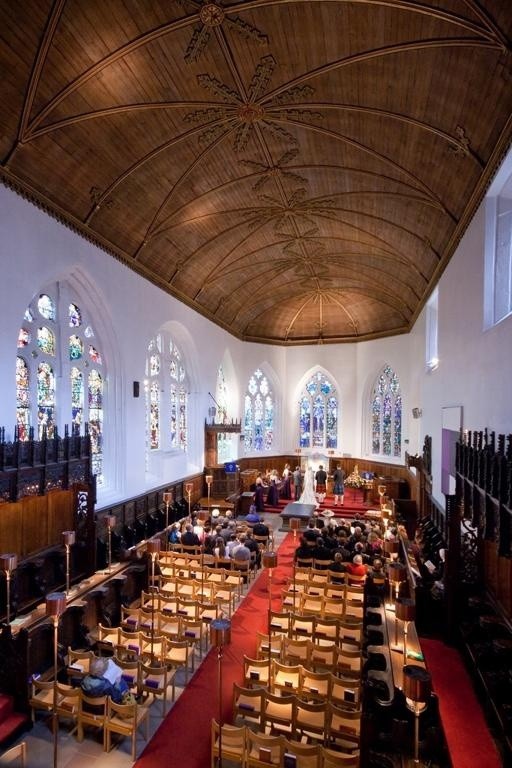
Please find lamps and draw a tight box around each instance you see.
[206,476,213,513]
[378,485,432,768]
[328,451,335,481]
[103,515,117,566]
[163,493,173,551]
[296,448,301,467]
[186,483,193,522]
[413,408,422,419]
[208,406,217,418]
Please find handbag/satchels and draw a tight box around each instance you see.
[120,690,138,719]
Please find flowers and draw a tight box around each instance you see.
[343,471,365,488]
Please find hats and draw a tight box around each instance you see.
[373,559,382,569]
[321,510,334,516]
[90,657,108,677]
[212,509,219,517]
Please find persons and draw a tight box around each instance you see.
[169,464,401,596]
[81,660,123,715]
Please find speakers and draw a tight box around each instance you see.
[211,407,216,417]
[413,408,418,418]
